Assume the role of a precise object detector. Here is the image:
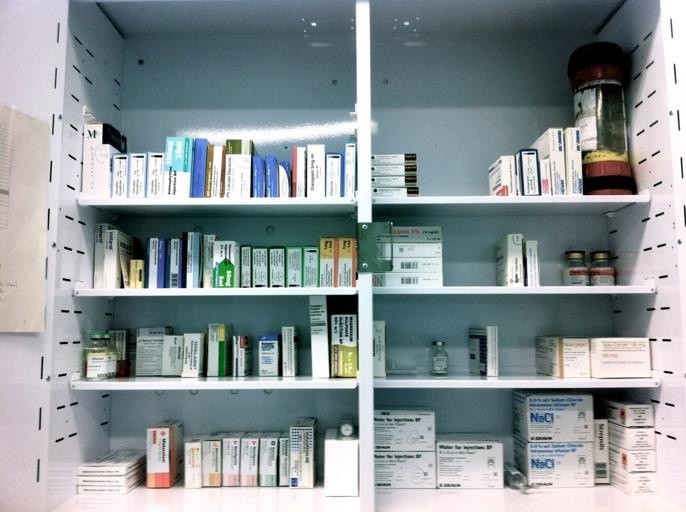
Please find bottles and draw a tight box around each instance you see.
[430,341,449,376]
[504,461,530,494]
[564,252,591,287]
[83,331,118,382]
[588,250,617,286]
[336,415,356,441]
[567,42,637,196]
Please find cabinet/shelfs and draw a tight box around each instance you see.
[2,2,677,504]
